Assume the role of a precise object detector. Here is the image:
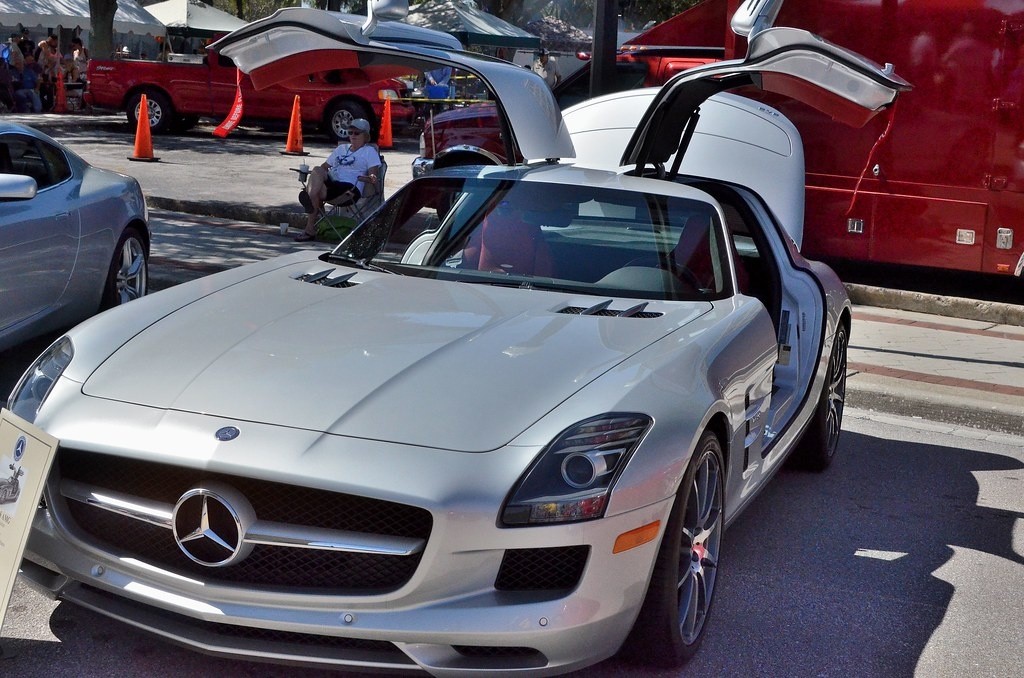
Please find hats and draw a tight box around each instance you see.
[347,118,370,133]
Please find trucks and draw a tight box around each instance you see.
[426,2,1024,291]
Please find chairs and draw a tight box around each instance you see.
[447,186,553,276]
[657,211,751,296]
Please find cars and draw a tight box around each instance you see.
[1,111,151,361]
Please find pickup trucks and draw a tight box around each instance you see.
[80,41,422,145]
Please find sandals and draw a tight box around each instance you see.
[298,191,314,214]
[294,231,315,242]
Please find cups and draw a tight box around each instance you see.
[298,164,309,182]
[280,223,288,235]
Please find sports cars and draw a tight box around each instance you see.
[13,0,913,678]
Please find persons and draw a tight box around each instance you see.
[294,118,383,242]
[530,48,562,89]
[422,67,452,117]
[0,27,208,113]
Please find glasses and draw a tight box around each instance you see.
[347,131,363,135]
